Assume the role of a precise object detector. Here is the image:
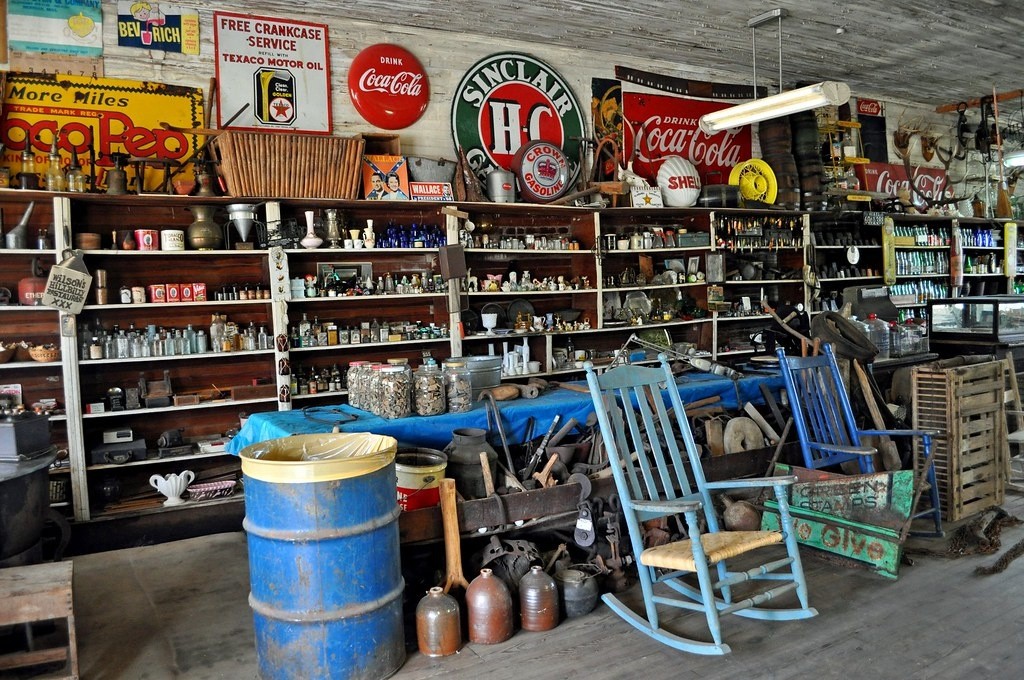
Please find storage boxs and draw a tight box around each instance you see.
[908,358,1005,520]
[0,414,51,461]
[193,283,206,301]
[148,284,166,303]
[165,284,179,302]
[180,283,192,302]
[356,133,400,157]
[925,294,1024,341]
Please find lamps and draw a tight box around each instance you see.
[1002,150,1024,167]
[697,8,851,137]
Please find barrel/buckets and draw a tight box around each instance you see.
[445,355,503,401]
[236,432,407,672]
[759,110,828,212]
[395,446,448,512]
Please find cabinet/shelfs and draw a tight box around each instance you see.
[0,186,1024,546]
[815,106,872,211]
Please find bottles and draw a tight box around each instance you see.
[488,337,530,376]
[823,132,860,190]
[413,364,446,416]
[847,308,927,361]
[880,222,1024,305]
[291,312,450,348]
[327,272,443,297]
[465,568,514,645]
[518,566,559,632]
[183,205,223,250]
[553,566,600,618]
[209,311,273,353]
[77,316,207,361]
[459,228,579,250]
[501,270,590,291]
[347,358,412,419]
[300,210,323,249]
[442,361,472,413]
[566,337,575,361]
[21,129,38,188]
[442,428,499,498]
[45,135,66,192]
[363,219,447,248]
[559,318,591,331]
[291,361,347,396]
[415,587,463,657]
[66,145,87,193]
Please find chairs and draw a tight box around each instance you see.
[777,341,947,539]
[582,353,820,655]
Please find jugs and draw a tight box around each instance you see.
[533,316,546,326]
[643,230,676,249]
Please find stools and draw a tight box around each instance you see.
[0,558,81,680]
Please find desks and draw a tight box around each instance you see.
[241,354,848,455]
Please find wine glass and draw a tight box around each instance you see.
[481,314,498,335]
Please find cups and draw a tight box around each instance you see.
[574,362,583,368]
[160,229,185,250]
[528,361,542,373]
[575,348,590,361]
[133,229,159,250]
[354,239,364,249]
[349,230,360,240]
[344,239,352,249]
[630,236,644,249]
[515,367,522,375]
[319,289,326,297]
[617,240,629,249]
[484,280,499,290]
[307,286,319,298]
